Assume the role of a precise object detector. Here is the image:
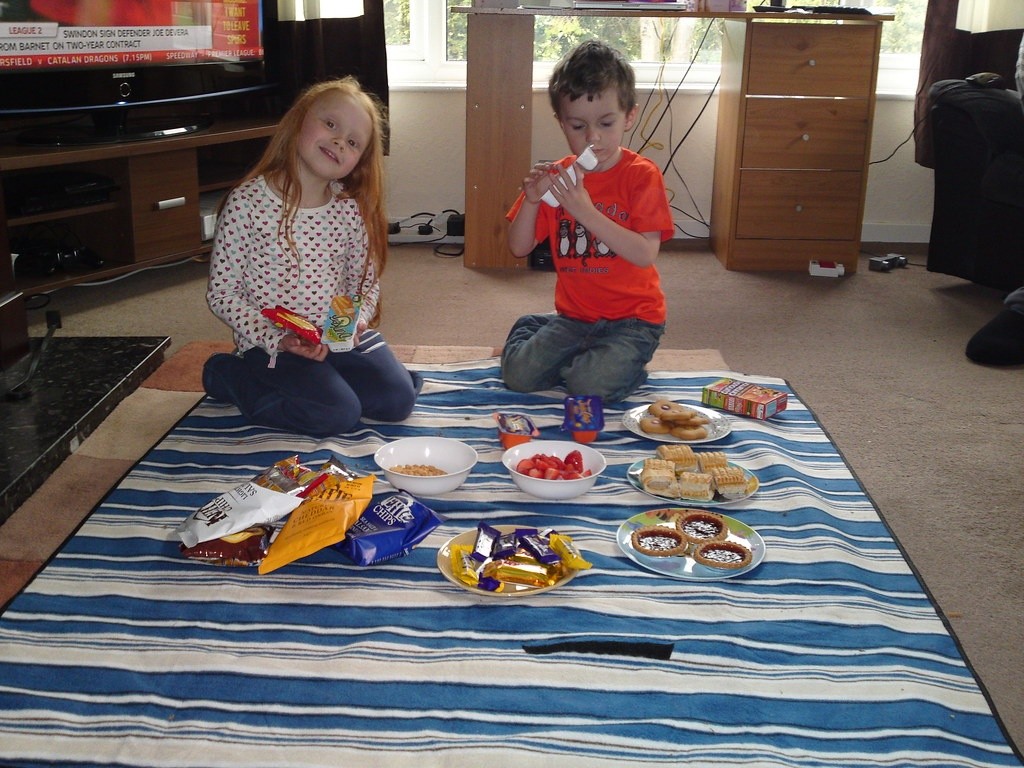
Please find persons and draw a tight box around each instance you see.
[500,41,674,405]
[202,77,416,435]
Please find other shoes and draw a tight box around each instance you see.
[966,309,1024,369]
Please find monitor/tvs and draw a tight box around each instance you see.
[0,0,281,146]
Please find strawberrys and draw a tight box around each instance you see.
[516,450,592,480]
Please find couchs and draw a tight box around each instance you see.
[923,79,1024,299]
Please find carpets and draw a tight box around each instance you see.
[0,341,731,614]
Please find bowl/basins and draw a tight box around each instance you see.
[374,436,479,495]
[501,441,607,499]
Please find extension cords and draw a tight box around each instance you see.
[387,227,464,244]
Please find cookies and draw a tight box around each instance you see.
[640,399,710,440]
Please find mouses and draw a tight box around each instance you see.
[965,73,1008,91]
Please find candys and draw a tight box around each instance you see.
[450,521,592,591]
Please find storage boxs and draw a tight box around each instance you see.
[702,378,788,420]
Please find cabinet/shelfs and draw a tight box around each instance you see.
[0,117,285,297]
[451,7,895,272]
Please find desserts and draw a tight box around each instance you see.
[638,444,747,498]
[631,512,753,573]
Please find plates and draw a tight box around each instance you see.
[623,404,733,442]
[616,511,768,584]
[626,457,758,509]
[436,525,577,598]
